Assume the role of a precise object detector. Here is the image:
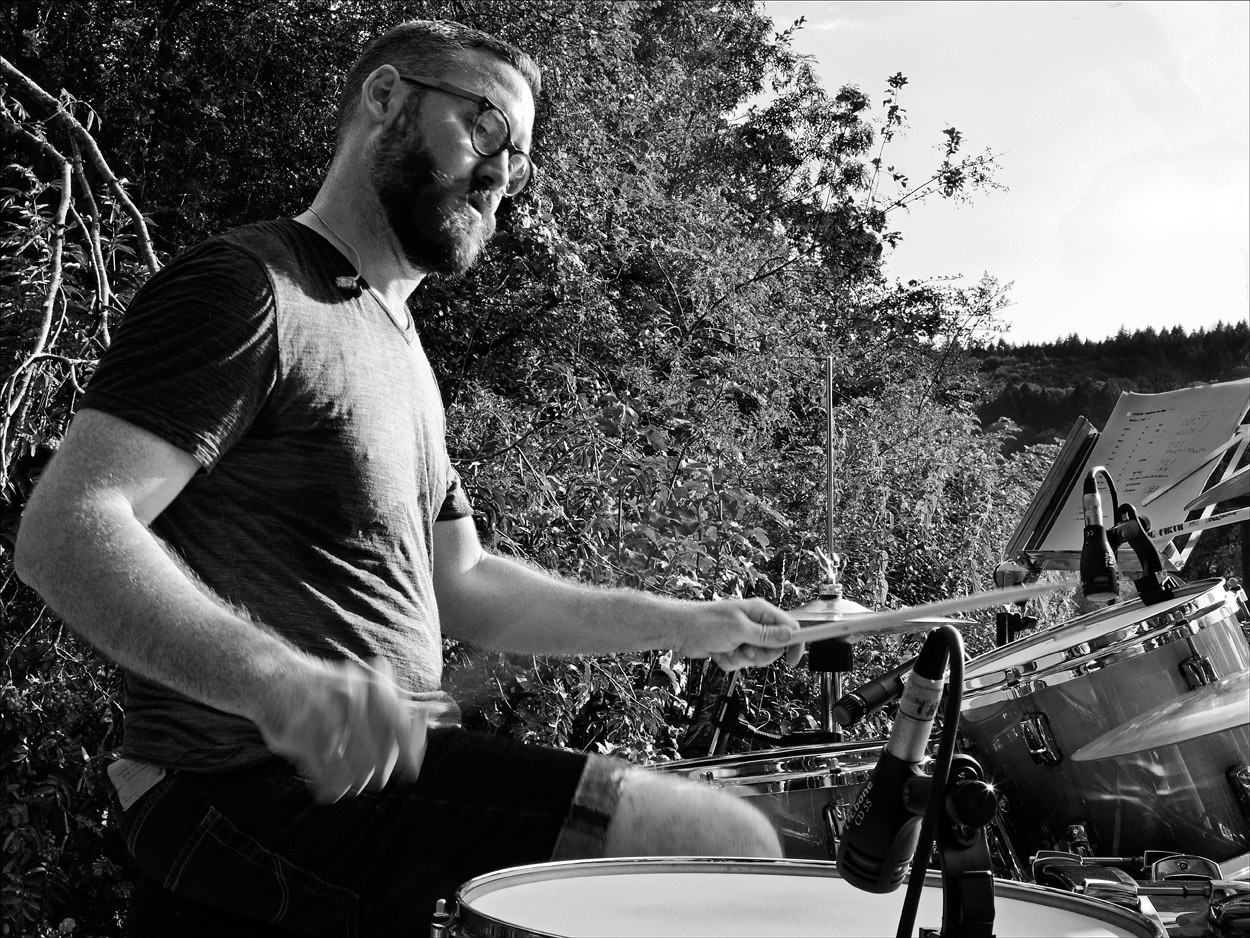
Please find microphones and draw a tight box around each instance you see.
[832,654,923,729]
[835,626,949,894]
[1079,477,1121,603]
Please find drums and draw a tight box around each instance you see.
[426,853,1173,938]
[955,574,1250,882]
[640,740,889,862]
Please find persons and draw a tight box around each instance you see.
[12,18,808,938]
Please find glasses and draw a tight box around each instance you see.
[400,74,535,198]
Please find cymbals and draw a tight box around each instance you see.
[1069,665,1249,763]
[787,599,980,635]
